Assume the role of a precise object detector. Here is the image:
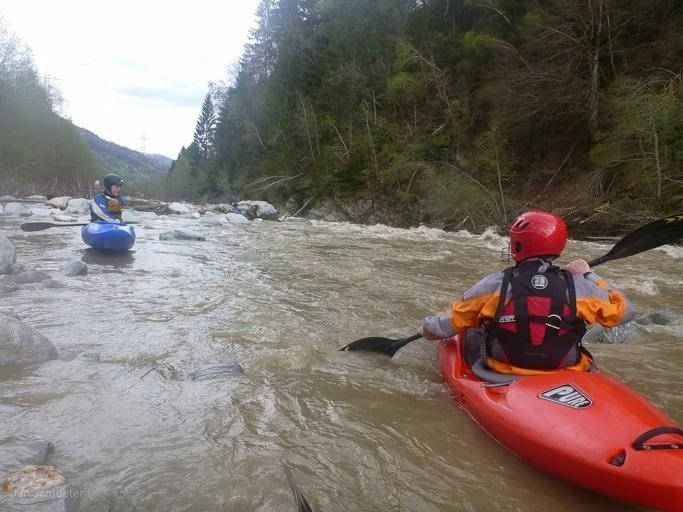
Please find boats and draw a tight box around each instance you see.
[80,218,135,251]
[435,300,682,512]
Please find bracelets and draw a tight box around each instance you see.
[580,268,594,279]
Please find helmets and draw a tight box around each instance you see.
[500,209,566,263]
[104,174,125,198]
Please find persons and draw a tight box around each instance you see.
[87,174,126,224]
[414,209,635,371]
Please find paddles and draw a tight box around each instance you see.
[337,214,683,359]
[19,221,138,231]
